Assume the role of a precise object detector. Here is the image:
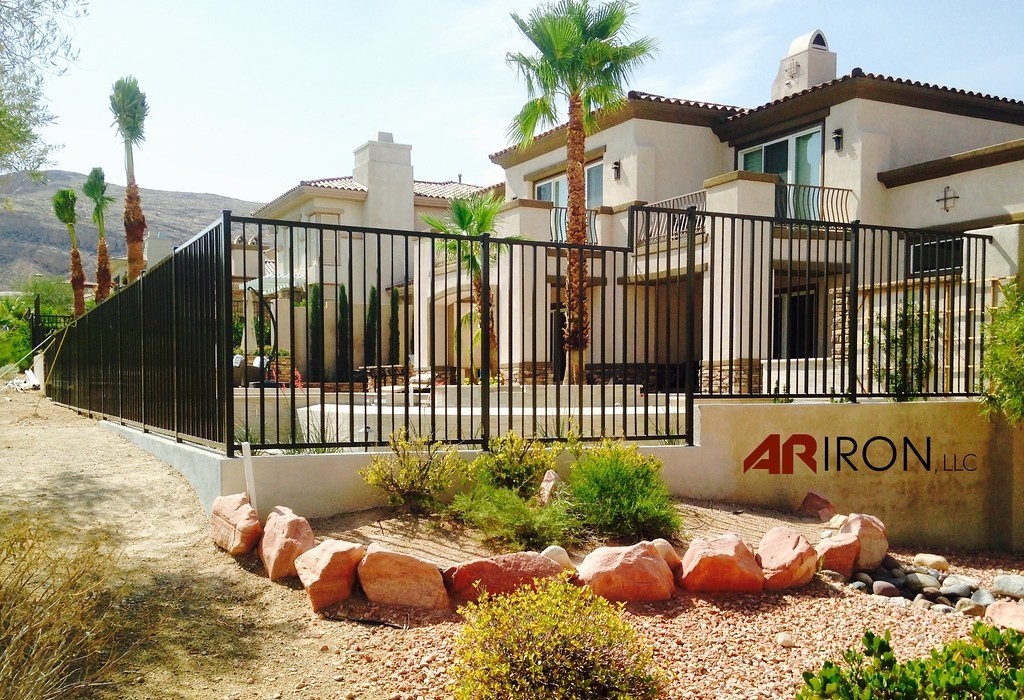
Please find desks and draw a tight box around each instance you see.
[357,364,407,393]
